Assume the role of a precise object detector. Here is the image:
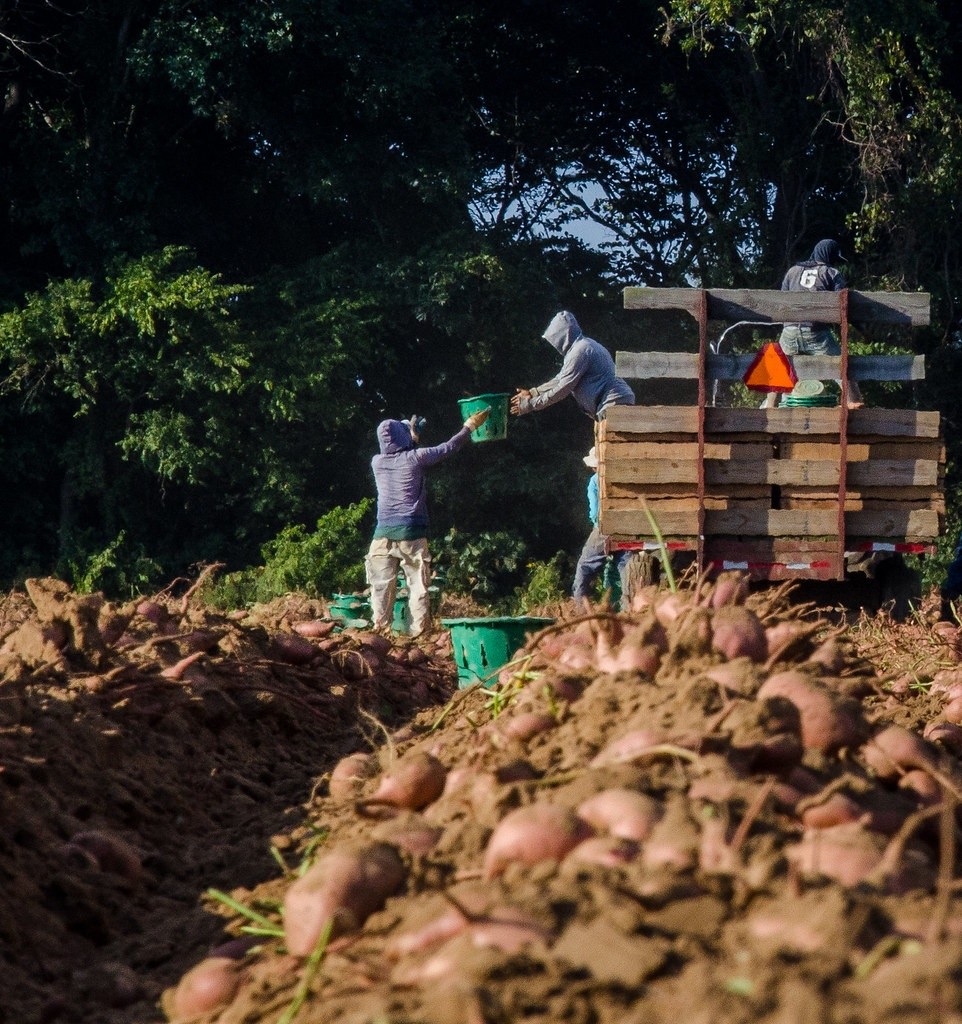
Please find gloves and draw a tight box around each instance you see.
[408,415,426,441]
[463,411,487,432]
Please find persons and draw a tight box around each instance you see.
[510,311,635,448]
[365,409,489,634]
[572,446,633,611]
[759,239,866,409]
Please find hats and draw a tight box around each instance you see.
[583,447,598,468]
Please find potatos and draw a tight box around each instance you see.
[0,573,962,1024]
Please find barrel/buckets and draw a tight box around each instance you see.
[786,380,838,408]
[440,615,558,689]
[457,393,513,443]
[321,570,444,635]
